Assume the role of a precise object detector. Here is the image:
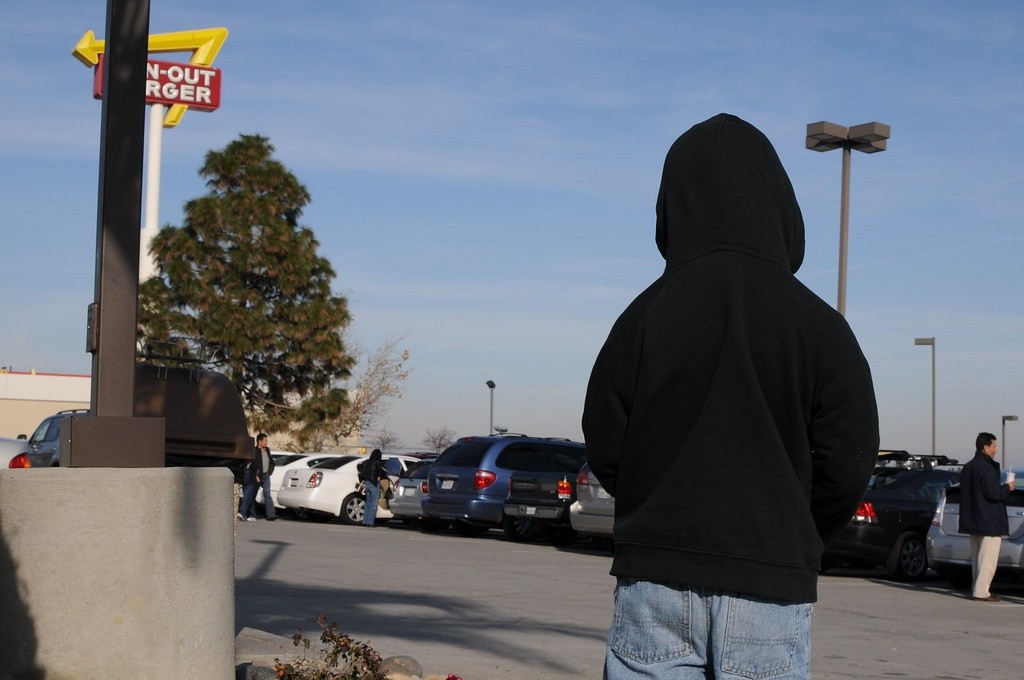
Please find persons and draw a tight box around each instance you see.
[958,433,1015,601]
[17,434,27,439]
[581,113,880,680]
[363,449,387,527]
[237,433,279,521]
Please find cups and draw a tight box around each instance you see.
[1005,472,1015,483]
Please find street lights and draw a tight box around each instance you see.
[804,120,892,314]
[486,380,497,437]
[915,338,937,454]
[1001,415,1019,469]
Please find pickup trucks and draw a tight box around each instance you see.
[504,469,594,549]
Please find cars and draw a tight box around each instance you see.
[243,449,420,523]
[834,466,964,582]
[0,437,28,472]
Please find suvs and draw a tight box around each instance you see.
[385,455,452,530]
[17,407,89,467]
[926,471,1024,592]
[421,435,589,541]
[570,463,617,546]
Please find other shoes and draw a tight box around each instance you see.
[238,513,256,522]
[266,516,279,521]
[362,523,377,528]
[973,592,1000,602]
